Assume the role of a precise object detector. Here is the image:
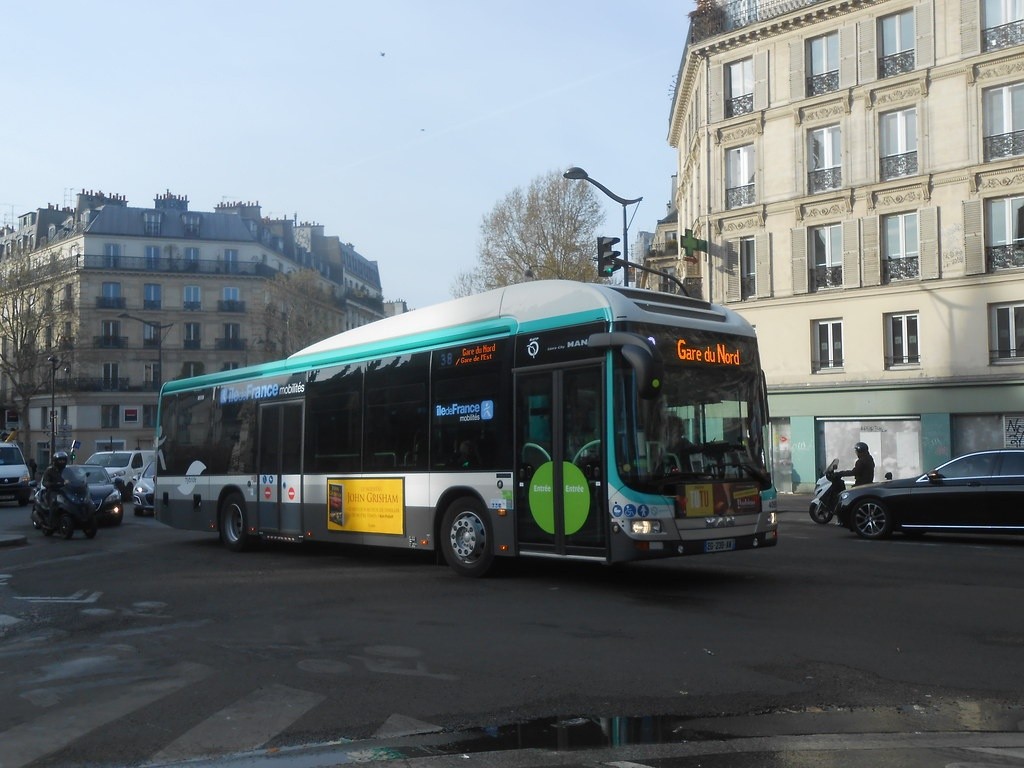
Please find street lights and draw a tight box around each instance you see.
[118,313,174,398]
[564,166,644,288]
[47,353,70,467]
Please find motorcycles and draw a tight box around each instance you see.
[31,465,99,538]
[808,457,891,525]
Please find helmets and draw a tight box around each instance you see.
[855,442,868,458]
[53,452,68,468]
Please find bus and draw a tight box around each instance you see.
[153,277,778,580]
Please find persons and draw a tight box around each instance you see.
[666,416,696,472]
[42,451,68,534]
[30,455,37,480]
[833,442,875,488]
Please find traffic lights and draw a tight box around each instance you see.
[597,236,622,277]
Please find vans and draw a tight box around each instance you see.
[85,450,155,502]
[0,441,31,507]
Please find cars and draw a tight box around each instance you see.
[838,449,1024,541]
[132,460,155,518]
[33,465,123,527]
